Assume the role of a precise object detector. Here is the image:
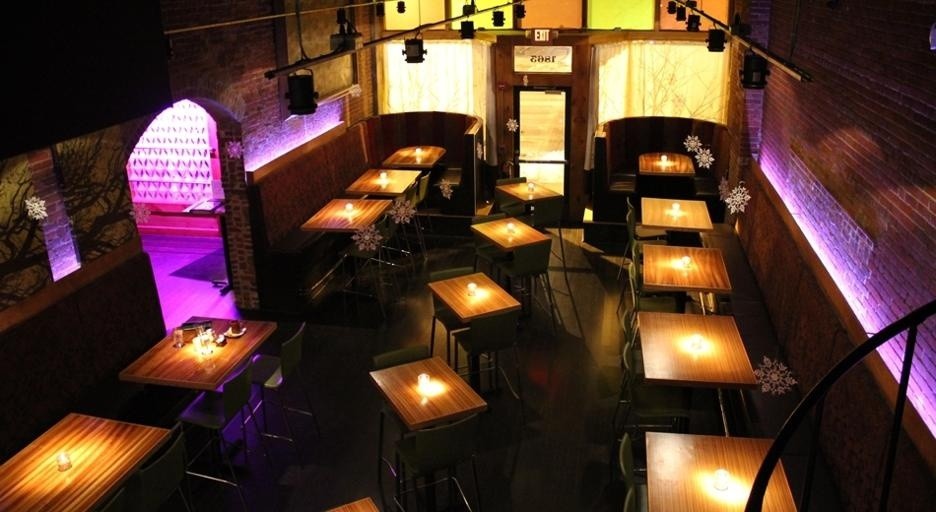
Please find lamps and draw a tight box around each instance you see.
[265,1,524,115]
[666,0,813,88]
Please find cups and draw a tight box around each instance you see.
[416,181,535,393]
[345,148,422,212]
[55,453,72,471]
[174,329,184,348]
[660,153,731,489]
[193,323,216,355]
[230,319,241,334]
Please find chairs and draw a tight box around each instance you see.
[616,198,636,512]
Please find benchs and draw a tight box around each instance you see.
[594,116,732,247]
[697,157,936,512]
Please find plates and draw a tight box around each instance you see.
[224,326,247,338]
